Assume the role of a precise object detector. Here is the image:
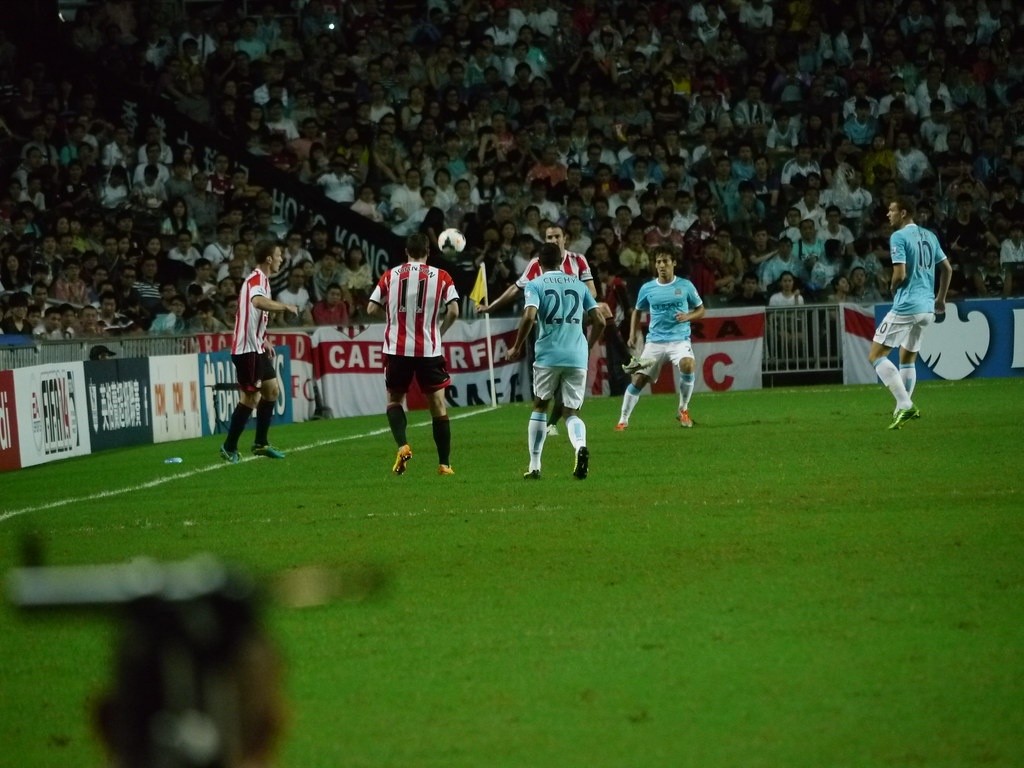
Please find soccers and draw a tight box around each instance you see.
[438,228,466,256]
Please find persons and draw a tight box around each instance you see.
[502,242,607,480]
[219,239,299,464]
[312,282,353,325]
[0,0,1024,343]
[867,196,954,432]
[612,244,707,431]
[366,232,460,477]
[89,345,117,361]
[478,223,598,437]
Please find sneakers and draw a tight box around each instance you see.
[889,403,920,429]
[574,447,589,480]
[220,445,243,464]
[676,409,693,427]
[393,444,412,474]
[616,423,628,432]
[546,424,560,436]
[523,469,541,480]
[439,465,454,474]
[252,443,285,458]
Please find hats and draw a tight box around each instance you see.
[90,346,116,358]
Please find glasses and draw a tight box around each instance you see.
[171,303,184,308]
[162,288,177,293]
